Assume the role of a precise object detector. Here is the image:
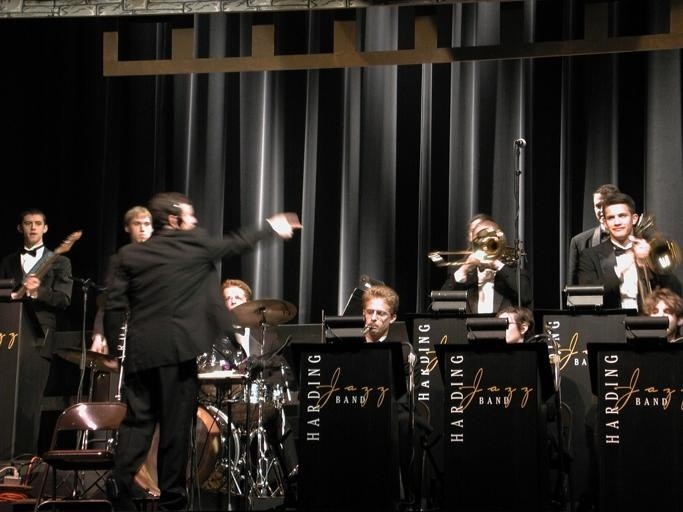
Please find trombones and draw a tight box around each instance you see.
[427,228,522,268]
[633,234,680,315]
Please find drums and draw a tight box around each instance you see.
[134,402,241,498]
[208,378,275,429]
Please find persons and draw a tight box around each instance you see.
[565,182,620,286]
[215,277,300,482]
[463,213,489,252]
[575,192,681,314]
[433,220,536,313]
[101,191,305,509]
[642,287,682,345]
[488,305,544,341]
[88,204,154,355]
[358,284,430,510]
[0,208,74,459]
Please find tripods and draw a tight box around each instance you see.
[55,286,286,500]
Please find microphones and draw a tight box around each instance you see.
[511,137,526,148]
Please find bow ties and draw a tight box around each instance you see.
[22,249,37,257]
[233,326,245,336]
[600,231,609,239]
[614,247,631,256]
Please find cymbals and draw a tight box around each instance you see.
[52,346,120,374]
[228,300,299,326]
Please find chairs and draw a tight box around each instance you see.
[33,399,126,511]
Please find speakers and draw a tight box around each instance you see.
[14,460,113,498]
